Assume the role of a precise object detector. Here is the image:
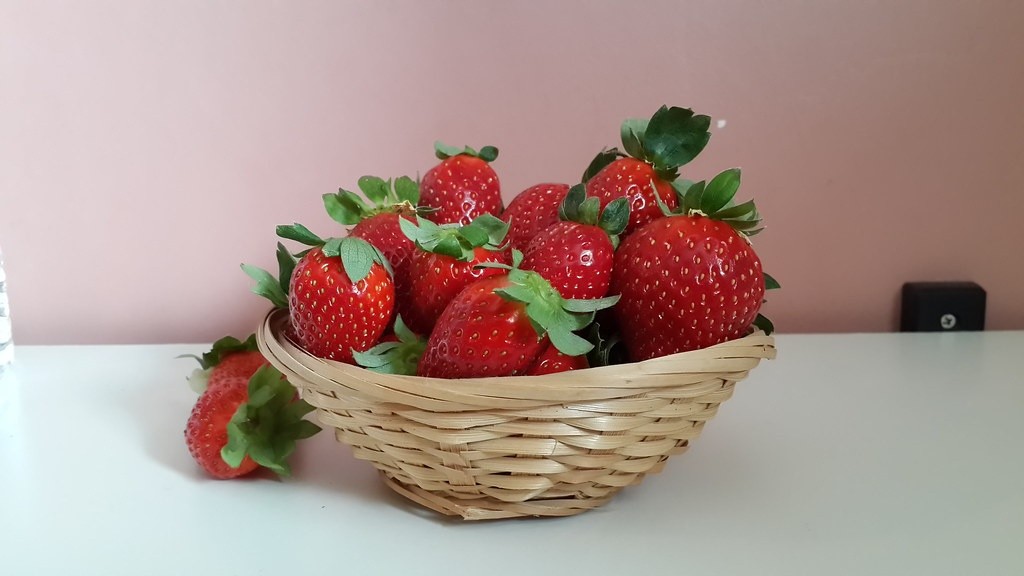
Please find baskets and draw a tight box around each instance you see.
[256,303,777,521]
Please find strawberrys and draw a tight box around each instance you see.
[183,333,325,479]
[238,105,781,381]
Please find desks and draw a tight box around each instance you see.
[0,332,1024,576]
